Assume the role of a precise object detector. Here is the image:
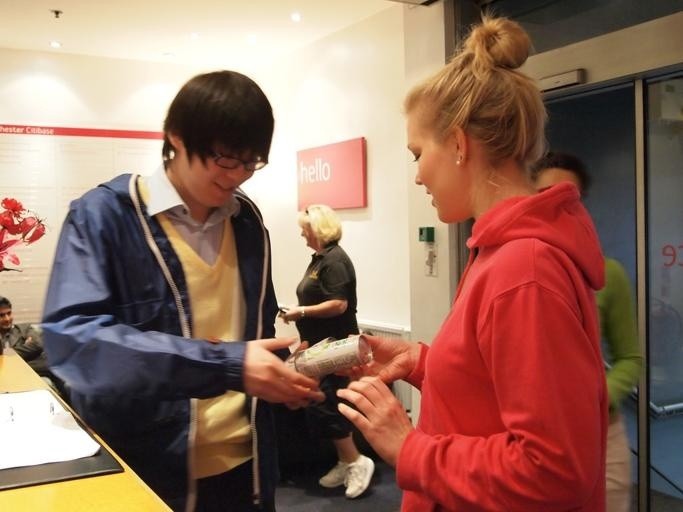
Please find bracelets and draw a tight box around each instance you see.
[301,306,305,318]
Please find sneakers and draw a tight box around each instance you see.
[318,454,375,499]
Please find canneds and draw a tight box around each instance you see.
[285,337,337,372]
[294,335,371,378]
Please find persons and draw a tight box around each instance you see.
[277,202,375,499]
[530,150,646,512]
[336,6,609,512]
[41,71,326,512]
[0,296,65,399]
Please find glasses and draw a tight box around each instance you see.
[205,147,267,171]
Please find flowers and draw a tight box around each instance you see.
[0,195,49,273]
